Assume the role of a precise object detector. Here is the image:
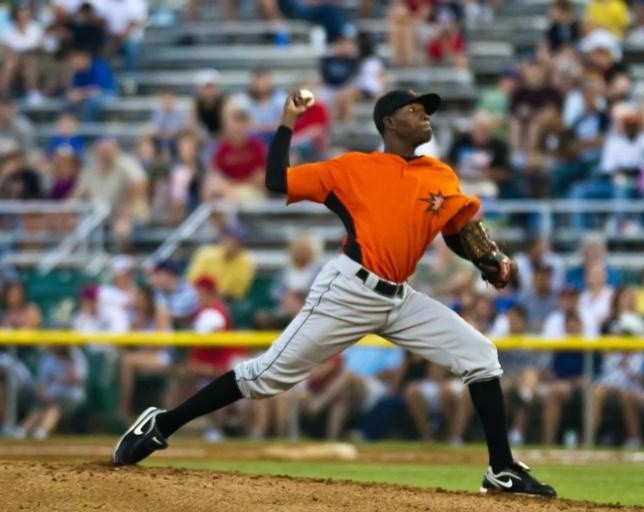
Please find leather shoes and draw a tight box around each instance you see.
[356,268,403,296]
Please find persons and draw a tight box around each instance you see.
[0,0,644,447]
[112,89,557,500]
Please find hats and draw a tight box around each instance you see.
[373,89,441,122]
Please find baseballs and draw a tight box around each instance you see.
[300,90,314,106]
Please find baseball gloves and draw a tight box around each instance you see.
[462,220,518,290]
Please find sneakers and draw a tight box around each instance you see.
[479,461,556,499]
[114,406,168,465]
